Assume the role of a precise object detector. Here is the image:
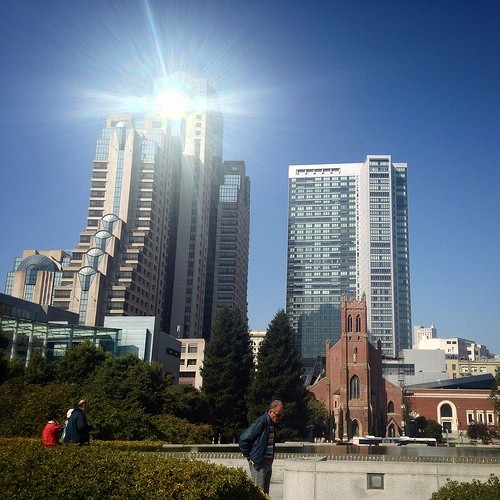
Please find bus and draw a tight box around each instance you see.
[353,434,437,448]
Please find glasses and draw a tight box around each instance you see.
[271,410,282,417]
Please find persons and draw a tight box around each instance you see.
[60,408,74,444]
[237,400,285,497]
[40,413,64,446]
[63,398,91,447]
